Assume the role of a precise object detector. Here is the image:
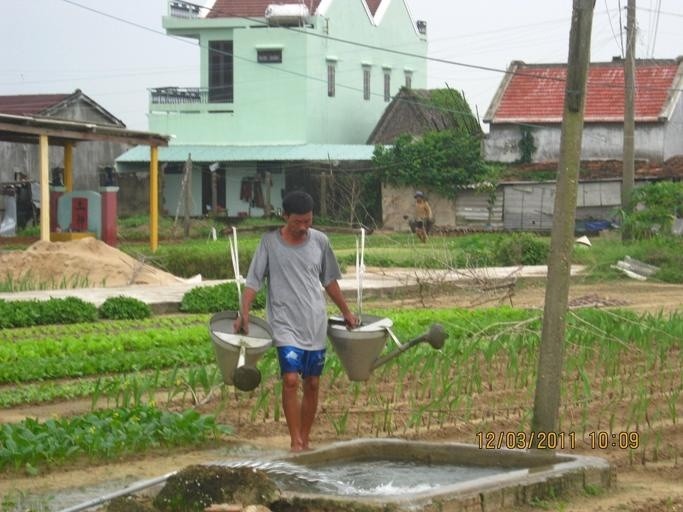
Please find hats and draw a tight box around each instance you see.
[414,191,424,198]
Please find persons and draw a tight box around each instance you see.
[414,191,433,243]
[400,214,411,233]
[234,191,358,452]
